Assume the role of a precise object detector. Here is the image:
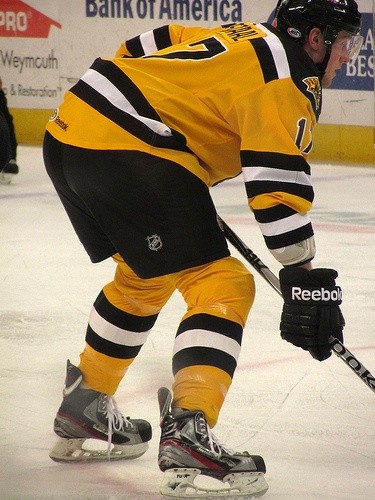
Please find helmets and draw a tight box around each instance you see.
[271,0,362,88]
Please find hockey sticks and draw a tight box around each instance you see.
[214,209,375,395]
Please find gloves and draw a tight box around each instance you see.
[278,265,346,362]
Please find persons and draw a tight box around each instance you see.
[43,1,362,499]
[0,80,20,174]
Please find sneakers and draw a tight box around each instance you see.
[49,358,152,461]
[155,387,270,500]
